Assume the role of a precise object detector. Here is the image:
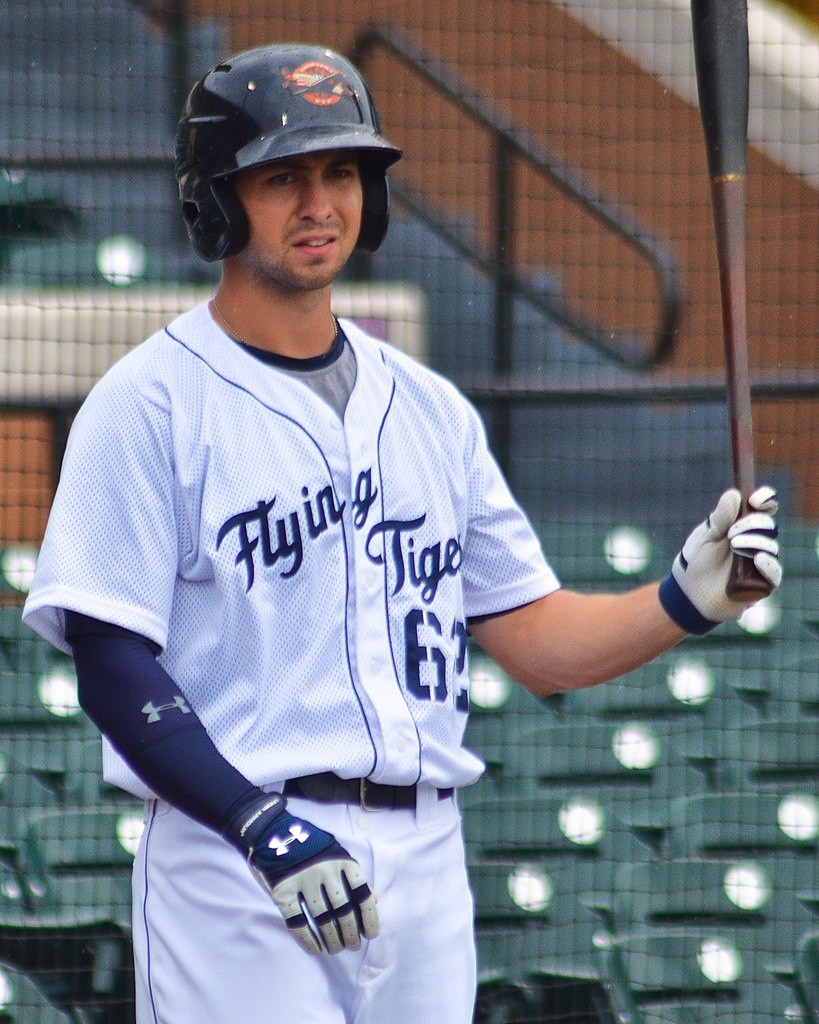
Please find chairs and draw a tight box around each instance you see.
[462,525,818,1023]
[0,603,141,1023]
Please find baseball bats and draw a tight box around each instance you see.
[690,1,773,606]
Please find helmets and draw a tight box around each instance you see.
[172,40,406,264]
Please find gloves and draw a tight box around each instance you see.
[657,484,783,638]
[217,786,381,957]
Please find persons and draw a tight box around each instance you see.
[20,45,783,1024]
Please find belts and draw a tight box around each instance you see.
[283,771,452,813]
